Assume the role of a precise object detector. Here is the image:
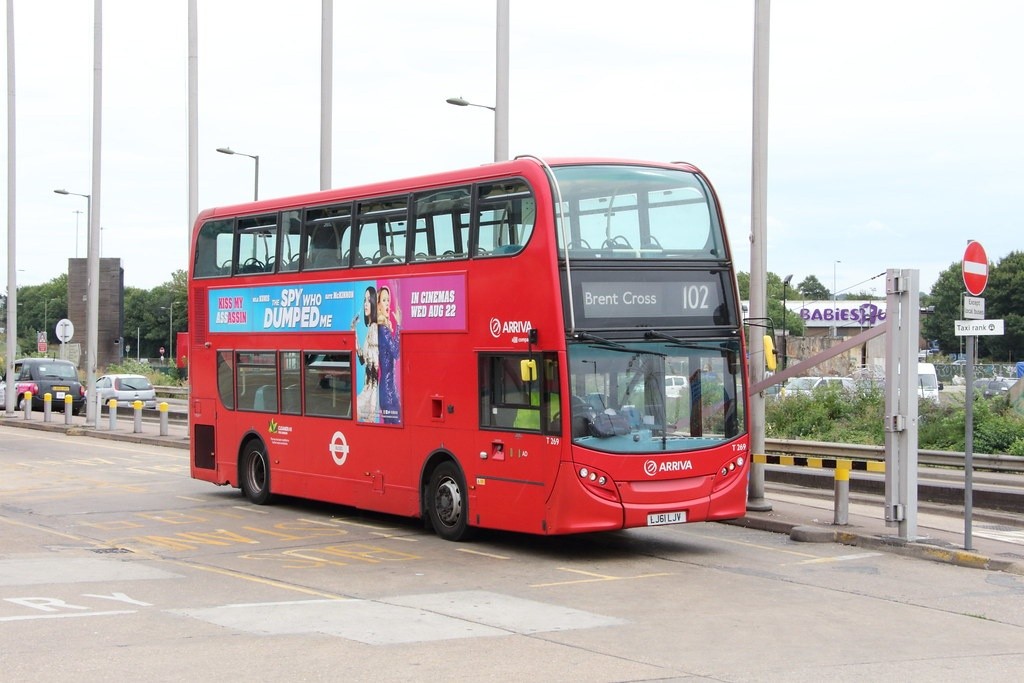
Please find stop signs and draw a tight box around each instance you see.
[159,346,165,355]
[961,242,989,296]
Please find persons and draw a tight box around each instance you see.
[351,286,402,425]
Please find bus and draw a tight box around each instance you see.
[190,156,779,545]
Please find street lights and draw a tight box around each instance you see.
[212,146,259,201]
[52,187,92,261]
[160,302,173,361]
[781,274,794,387]
[444,97,499,248]
[833,259,842,338]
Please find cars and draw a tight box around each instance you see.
[83,375,157,410]
[917,348,967,366]
[973,376,1020,400]
[0,358,86,416]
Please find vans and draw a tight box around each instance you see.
[774,377,857,404]
[631,375,686,398]
[899,362,944,405]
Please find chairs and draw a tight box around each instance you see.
[621,405,642,428]
[571,390,610,411]
[254,384,304,413]
[220,236,663,274]
